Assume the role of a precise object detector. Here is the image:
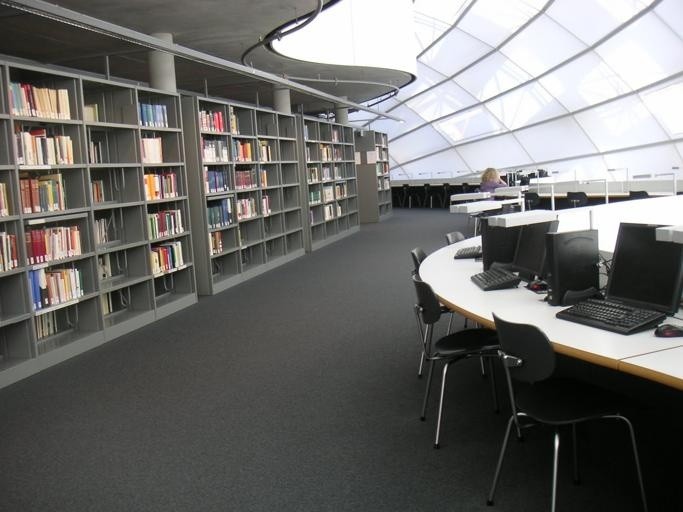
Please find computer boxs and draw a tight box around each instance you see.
[480,216,521,272]
[544,228,600,305]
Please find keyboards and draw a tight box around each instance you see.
[470,267,521,291]
[454,245,480,259]
[556,298,666,336]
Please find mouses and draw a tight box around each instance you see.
[531,281,548,291]
[654,323,683,338]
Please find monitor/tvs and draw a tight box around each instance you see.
[605,222,683,316]
[510,220,560,280]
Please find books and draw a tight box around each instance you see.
[323,185,334,203]
[205,230,225,256]
[96,253,115,282]
[1,231,20,272]
[92,216,109,248]
[0,180,10,217]
[255,139,273,163]
[149,240,184,276]
[305,123,309,140]
[231,138,255,164]
[97,290,116,317]
[332,130,340,143]
[142,170,179,201]
[13,126,74,167]
[232,163,259,190]
[259,194,272,218]
[376,145,381,161]
[18,171,70,214]
[196,111,226,134]
[307,146,310,161]
[34,312,58,341]
[379,177,383,191]
[384,176,390,188]
[84,103,103,125]
[22,223,82,263]
[336,201,344,216]
[324,204,334,222]
[85,125,104,165]
[318,143,333,161]
[145,209,184,240]
[205,198,235,228]
[236,196,258,221]
[89,177,104,206]
[336,183,347,200]
[7,80,70,122]
[138,136,164,164]
[308,166,320,183]
[202,165,232,193]
[323,165,331,181]
[382,135,386,146]
[258,168,267,188]
[27,268,83,312]
[382,147,387,160]
[310,191,322,204]
[138,101,170,129]
[201,136,231,163]
[228,107,242,137]
[376,163,382,173]
[334,165,345,180]
[382,163,388,174]
[333,147,343,161]
[310,210,314,224]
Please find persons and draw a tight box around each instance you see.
[476,168,510,221]
